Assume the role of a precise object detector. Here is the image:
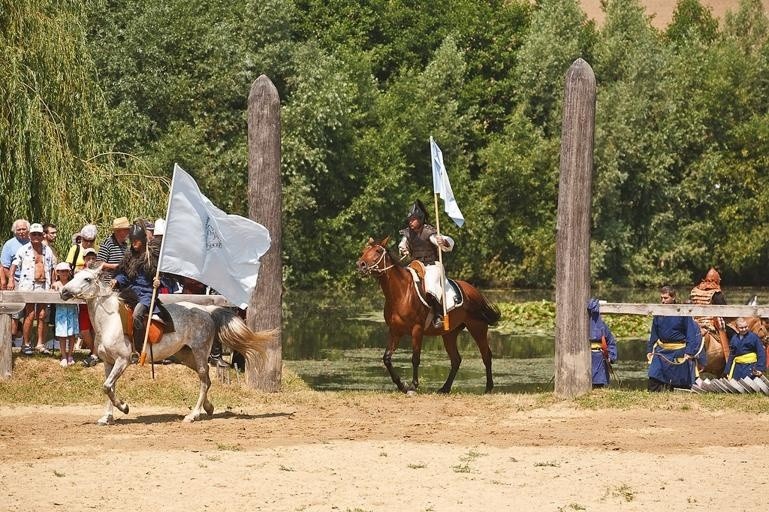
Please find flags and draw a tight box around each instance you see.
[157,163,272,310]
[429,135,466,229]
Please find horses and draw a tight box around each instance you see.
[356,236,502,398]
[59,257,281,425]
[692,317,769,379]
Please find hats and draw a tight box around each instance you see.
[29,217,166,274]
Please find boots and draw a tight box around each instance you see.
[431,296,443,329]
[130,315,145,364]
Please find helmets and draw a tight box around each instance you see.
[407,204,425,224]
[697,267,721,290]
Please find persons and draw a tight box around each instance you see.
[0,216,246,374]
[644,265,769,393]
[587,296,618,390]
[398,200,458,329]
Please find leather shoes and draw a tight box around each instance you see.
[212,357,231,367]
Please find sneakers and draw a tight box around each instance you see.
[13,336,101,367]
